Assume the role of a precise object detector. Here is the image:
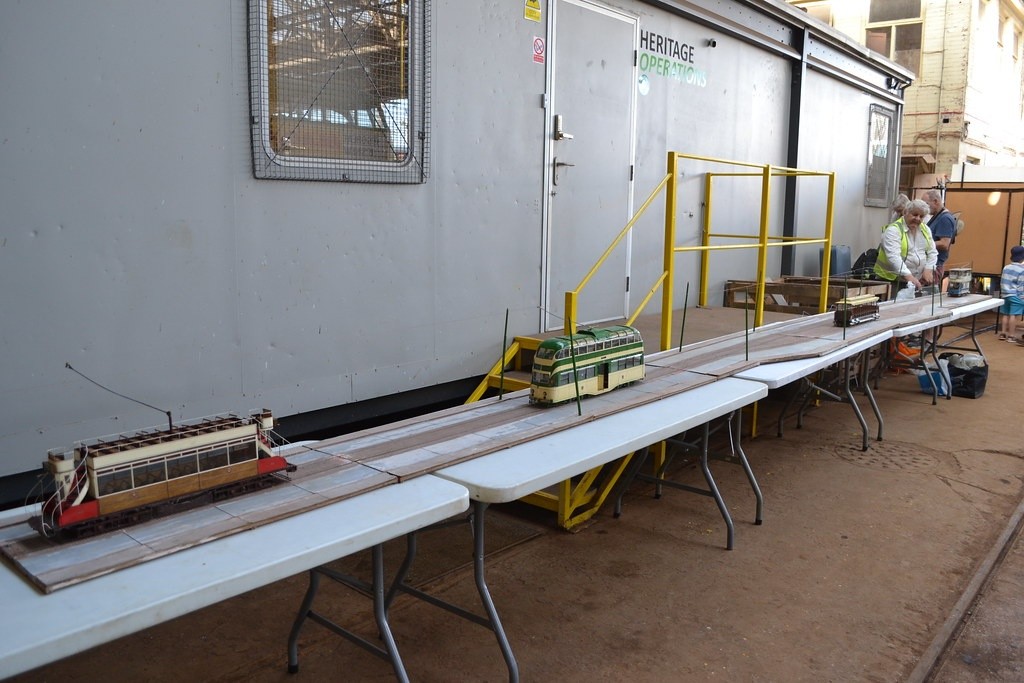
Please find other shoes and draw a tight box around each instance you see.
[893,334,934,355]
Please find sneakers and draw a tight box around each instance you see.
[1015,338,1024,347]
[1006,337,1021,342]
[998,333,1007,339]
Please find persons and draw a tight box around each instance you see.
[873,190,957,352]
[998,246,1024,342]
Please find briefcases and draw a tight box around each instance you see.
[818,244,851,277]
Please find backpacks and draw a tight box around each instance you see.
[851,248,879,279]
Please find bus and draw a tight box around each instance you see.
[529,324,647,407]
[947,268,973,296]
[833,294,880,328]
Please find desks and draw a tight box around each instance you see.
[870,288,1005,400]
[385,375,769,683]
[644,318,895,526]
[0,438,470,683]
[800,299,961,442]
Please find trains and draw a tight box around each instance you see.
[26,408,298,546]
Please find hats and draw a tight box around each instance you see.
[1010,246,1024,262]
